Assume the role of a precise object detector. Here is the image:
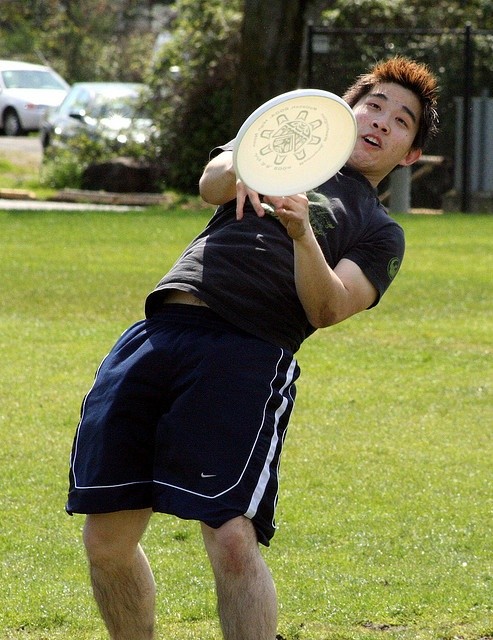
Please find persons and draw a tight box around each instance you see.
[65,52,441,640]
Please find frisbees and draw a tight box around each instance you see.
[231,89,358,198]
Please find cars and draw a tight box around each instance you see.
[0,60,71,136]
[41,83,149,148]
